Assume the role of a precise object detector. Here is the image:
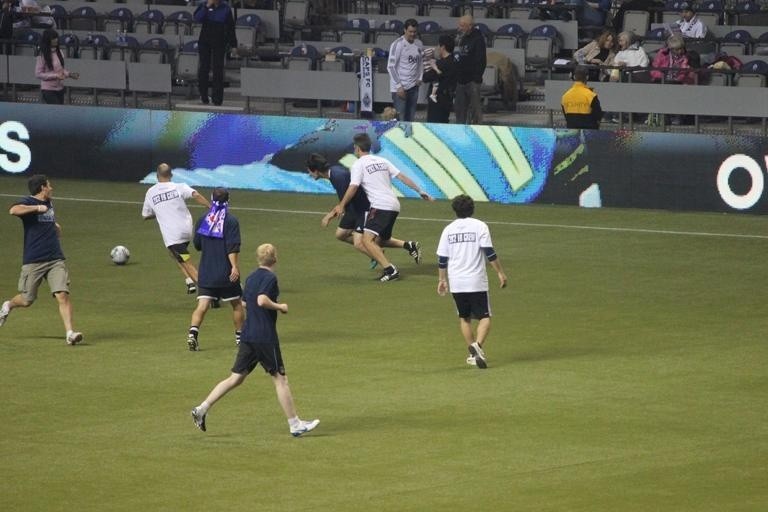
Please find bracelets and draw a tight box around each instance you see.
[37,205,42,212]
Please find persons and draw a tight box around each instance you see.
[0,174,83,346]
[320,133,434,283]
[418,46,442,104]
[192,0,238,107]
[386,18,424,122]
[140,162,213,294]
[35,29,80,104]
[572,0,709,84]
[422,35,458,123]
[561,66,602,129]
[188,186,245,352]
[436,194,508,369]
[191,243,321,438]
[455,15,487,124]
[305,152,385,270]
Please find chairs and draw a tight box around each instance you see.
[544,2,767,125]
[1,1,309,97]
[240,0,590,111]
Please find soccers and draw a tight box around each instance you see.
[112,244,131,266]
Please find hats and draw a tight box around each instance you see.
[679,2,693,11]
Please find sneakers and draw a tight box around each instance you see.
[66,331,83,346]
[368,246,385,269]
[0,300,10,327]
[409,240,422,264]
[290,419,321,437]
[210,297,221,309]
[191,406,207,432]
[186,333,199,353]
[466,341,489,370]
[374,271,398,282]
[600,113,683,126]
[429,95,437,103]
[187,281,197,294]
